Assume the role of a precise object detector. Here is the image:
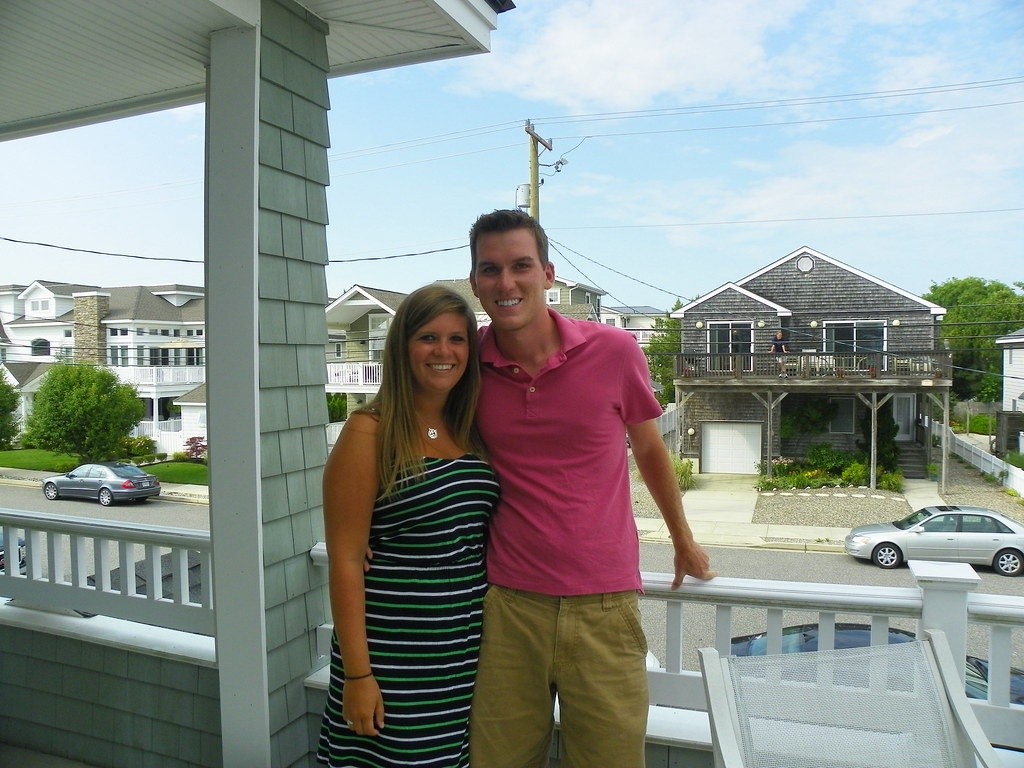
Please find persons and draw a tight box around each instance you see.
[768,331,790,379]
[315,283,502,768]
[362,209,717,768]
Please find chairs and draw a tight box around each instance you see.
[801,356,836,377]
[698,629,1003,768]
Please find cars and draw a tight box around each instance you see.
[0,533,27,575]
[42,462,162,506]
[844,506,1024,577]
[731,623,1024,705]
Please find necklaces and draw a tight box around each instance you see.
[413,407,449,441]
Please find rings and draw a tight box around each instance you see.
[346,720,354,725]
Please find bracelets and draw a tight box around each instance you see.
[344,672,373,680]
